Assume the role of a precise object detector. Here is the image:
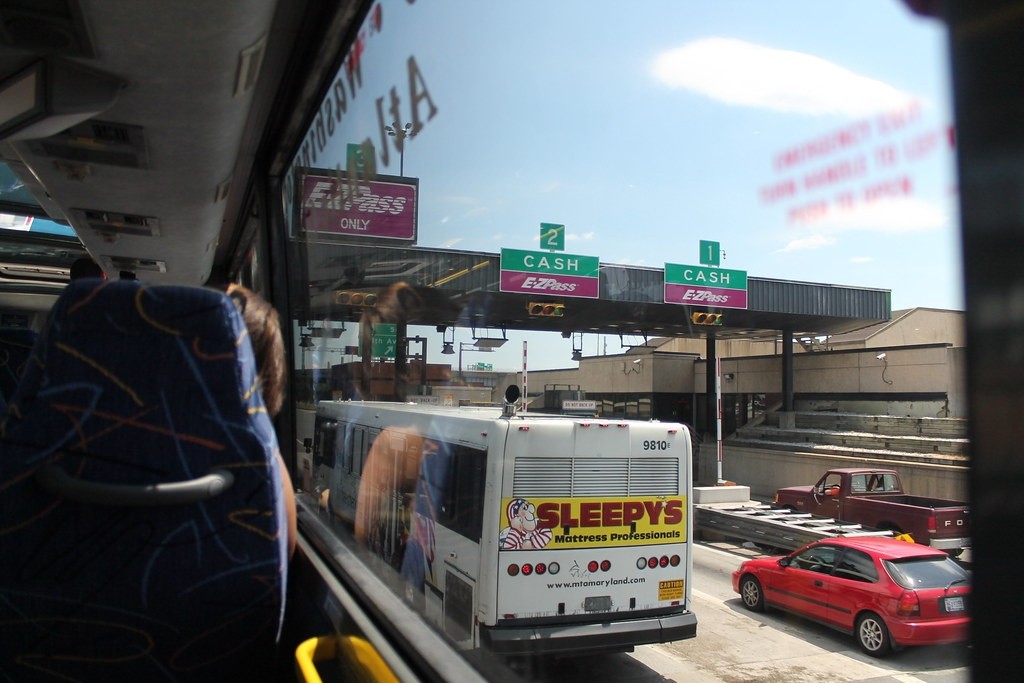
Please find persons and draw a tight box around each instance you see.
[349,278,436,578]
[212,280,299,562]
[69,256,108,281]
[824,488,841,496]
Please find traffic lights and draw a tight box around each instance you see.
[336,291,377,307]
[693,312,723,326]
[528,302,565,317]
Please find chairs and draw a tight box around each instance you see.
[1,276,285,683]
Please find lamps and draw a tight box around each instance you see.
[571,332,583,361]
[298,321,315,348]
[441,327,456,354]
[723,373,733,380]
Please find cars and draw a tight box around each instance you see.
[732,536,972,658]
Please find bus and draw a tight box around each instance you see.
[303,385,698,659]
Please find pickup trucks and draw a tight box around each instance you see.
[771,467,972,556]
